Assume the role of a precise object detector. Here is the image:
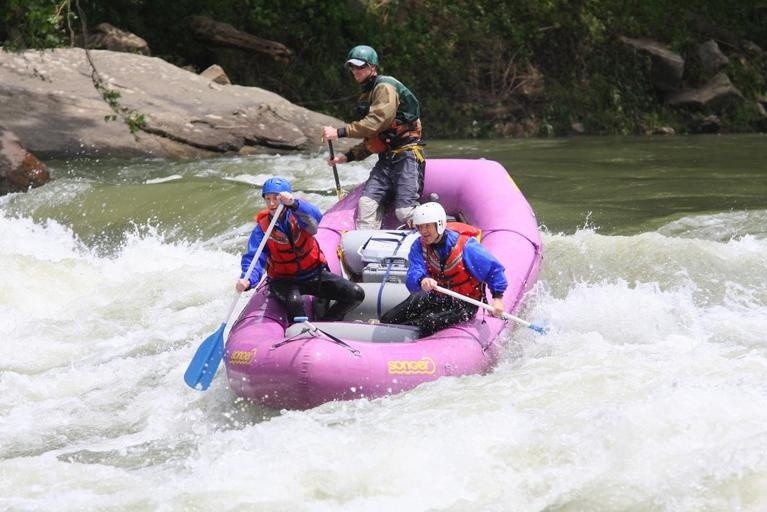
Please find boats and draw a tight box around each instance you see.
[224,157,543,410]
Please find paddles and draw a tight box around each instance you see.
[328,140,350,201]
[184,201,285,391]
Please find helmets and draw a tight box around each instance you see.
[412,201,447,234]
[262,178,292,197]
[343,45,378,69]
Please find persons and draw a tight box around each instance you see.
[380,202,508,338]
[319,46,426,231]
[234,177,364,326]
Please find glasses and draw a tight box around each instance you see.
[348,63,366,70]
